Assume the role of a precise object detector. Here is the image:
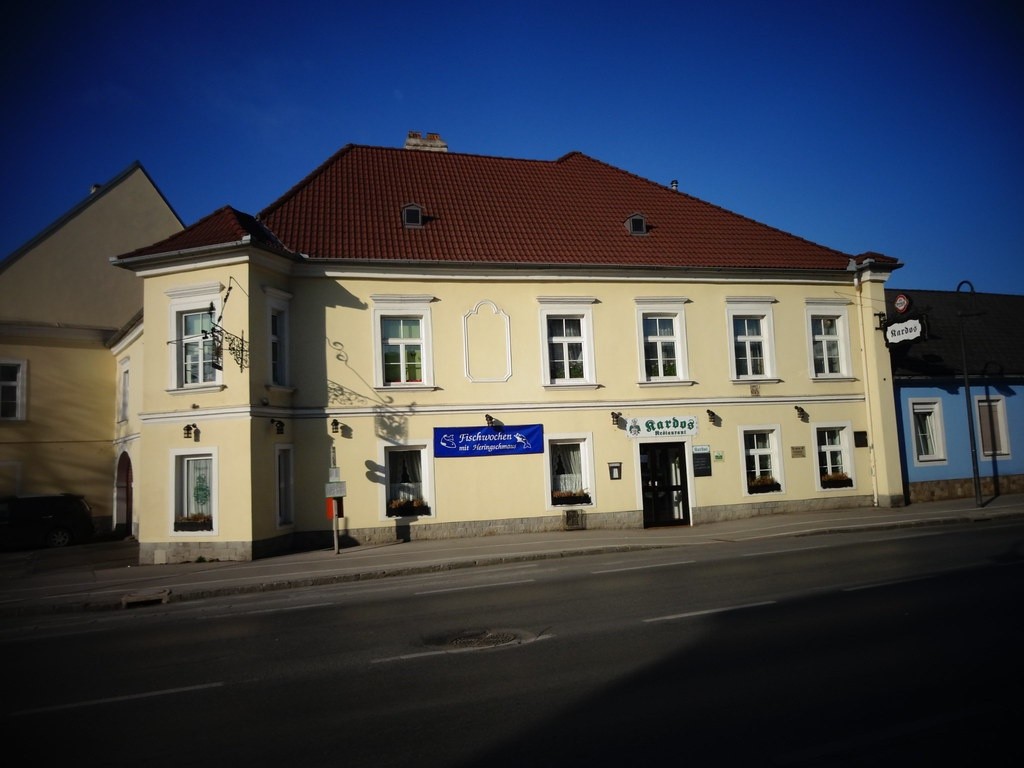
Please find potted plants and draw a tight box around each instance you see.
[551,490,591,504]
[386,497,431,515]
[821,471,852,488]
[748,475,782,493]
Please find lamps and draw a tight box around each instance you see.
[183,423,196,438]
[794,406,804,420]
[485,414,495,427]
[270,418,285,434]
[707,410,716,422]
[331,419,339,433]
[611,412,622,425]
